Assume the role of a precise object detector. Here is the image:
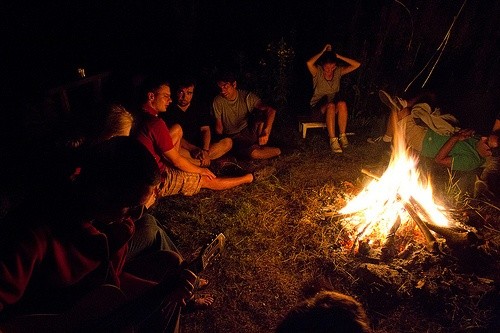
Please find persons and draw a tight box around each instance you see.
[133,83,277,198]
[366,52,500,171]
[0,137,217,333]
[66,105,159,212]
[210,79,305,160]
[276,290,365,333]
[159,78,233,167]
[305,44,361,154]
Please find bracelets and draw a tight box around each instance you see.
[202,149,209,154]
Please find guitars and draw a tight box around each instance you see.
[0,231,228,333]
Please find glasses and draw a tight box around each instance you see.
[484,136,491,148]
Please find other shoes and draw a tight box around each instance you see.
[379,89,404,112]
[251,165,276,182]
[339,132,350,148]
[367,135,391,144]
[330,136,343,153]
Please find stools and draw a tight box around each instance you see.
[297,116,328,139]
